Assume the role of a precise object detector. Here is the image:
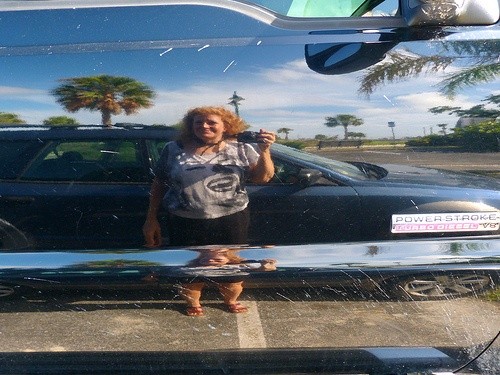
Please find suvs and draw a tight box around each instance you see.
[1,122,499,299]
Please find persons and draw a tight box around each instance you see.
[141,105,278,317]
[141,247,278,316]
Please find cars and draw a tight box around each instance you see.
[0,0,500,375]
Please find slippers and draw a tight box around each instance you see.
[186,305,203,316]
[224,300,248,313]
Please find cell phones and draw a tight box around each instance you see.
[236,131,263,143]
